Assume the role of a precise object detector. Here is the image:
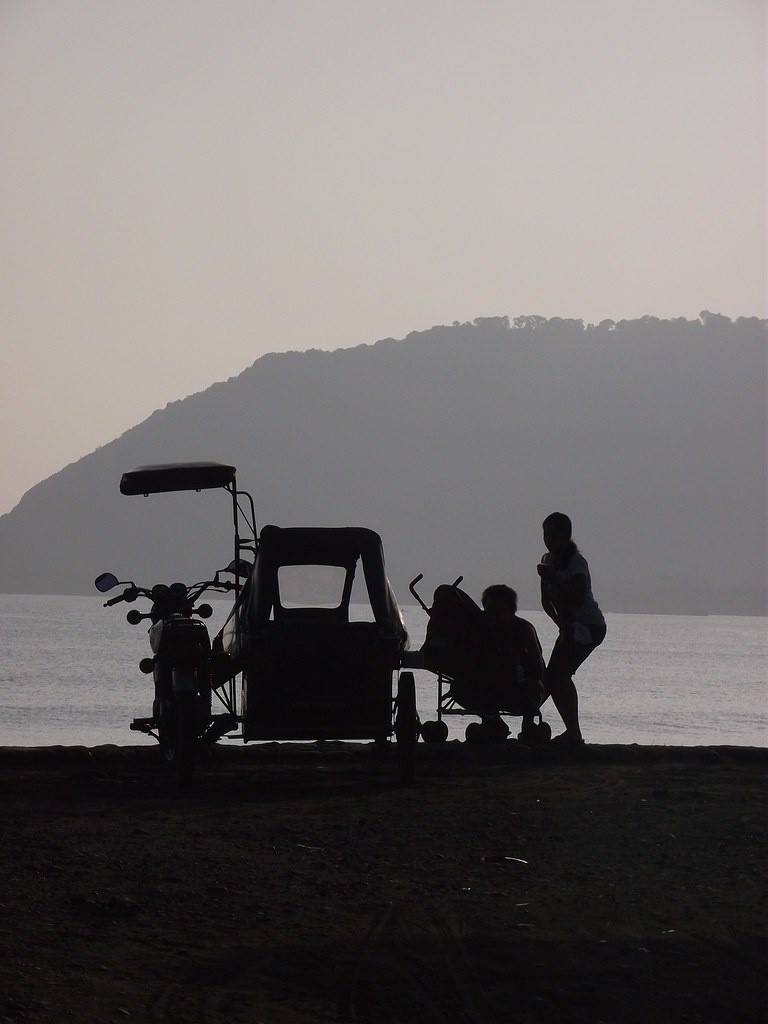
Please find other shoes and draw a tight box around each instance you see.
[554,733,584,747]
[499,720,536,741]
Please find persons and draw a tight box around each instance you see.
[537,512,607,742]
[449,585,553,740]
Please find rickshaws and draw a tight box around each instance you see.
[94,461,418,780]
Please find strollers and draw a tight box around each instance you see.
[408,573,552,746]
